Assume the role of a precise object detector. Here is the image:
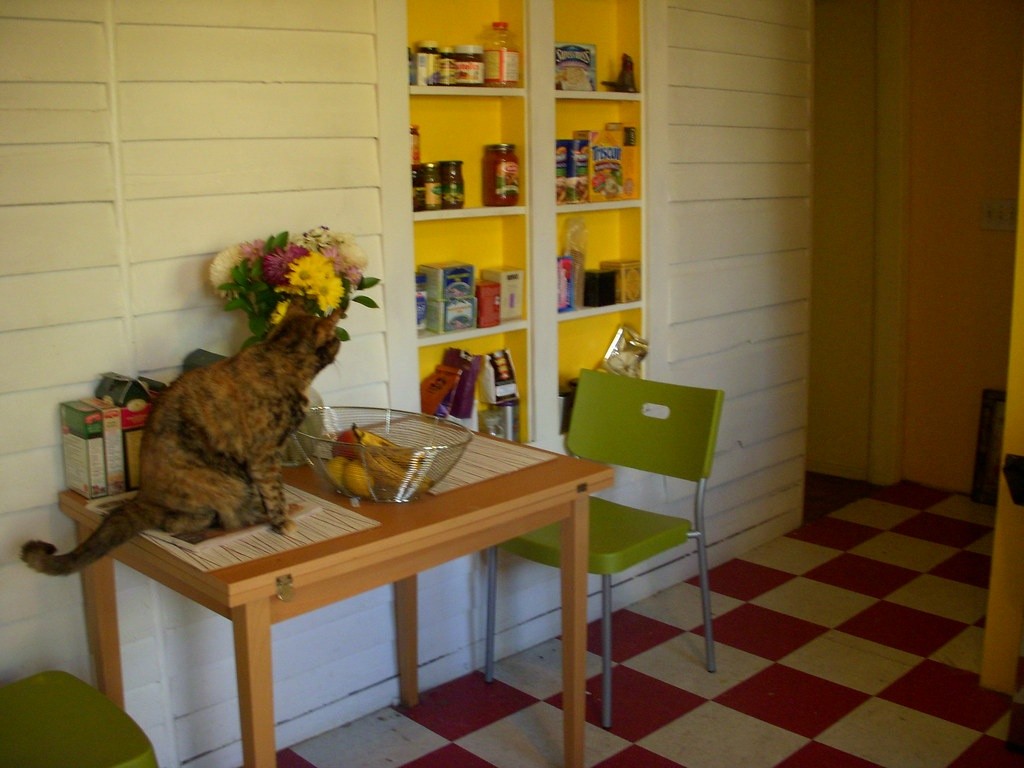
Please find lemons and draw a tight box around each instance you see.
[320,456,375,499]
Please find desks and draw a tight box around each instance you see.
[57,412,615,768]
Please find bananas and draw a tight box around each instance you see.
[352,424,436,490]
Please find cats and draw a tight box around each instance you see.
[17,293,341,577]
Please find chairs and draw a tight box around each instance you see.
[0,671,158,768]
[484,368,725,727]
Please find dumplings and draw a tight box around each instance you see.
[410,124,464,211]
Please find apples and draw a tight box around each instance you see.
[331,430,361,460]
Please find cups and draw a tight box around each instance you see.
[479,409,506,439]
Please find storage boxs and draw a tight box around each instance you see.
[420,122,642,332]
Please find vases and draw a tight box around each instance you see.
[278,385,326,467]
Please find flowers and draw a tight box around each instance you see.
[217,226,381,342]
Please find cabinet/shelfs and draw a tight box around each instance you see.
[406,1,645,445]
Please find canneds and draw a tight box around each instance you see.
[483,143,518,208]
[407,40,485,86]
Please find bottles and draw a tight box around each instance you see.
[481,20,519,87]
